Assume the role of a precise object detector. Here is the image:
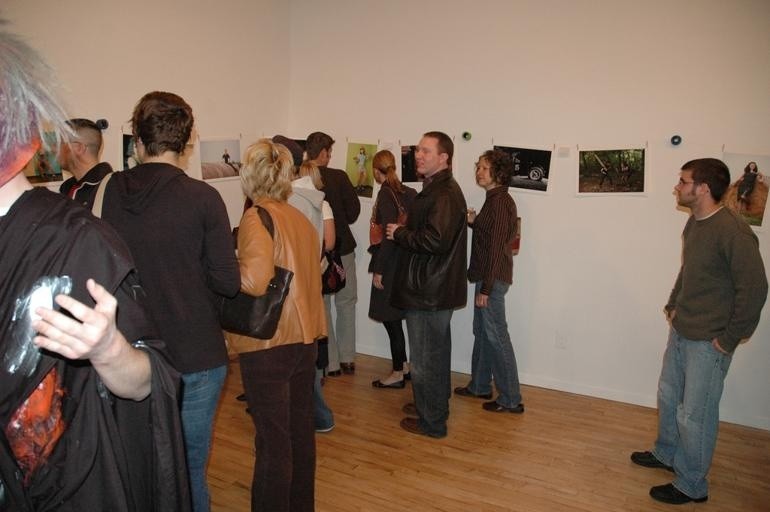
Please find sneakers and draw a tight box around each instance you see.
[631,450,674,469]
[649,483,708,503]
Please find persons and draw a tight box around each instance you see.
[235,133,361,432]
[512,152,520,176]
[386,132,467,438]
[454,150,525,413]
[55,116,113,209]
[0,16,197,511]
[83,90,242,511]
[621,163,632,188]
[353,145,372,191]
[224,138,328,512]
[629,156,768,505]
[367,150,418,389]
[731,160,764,215]
[598,162,614,189]
[38,152,56,180]
[221,148,232,164]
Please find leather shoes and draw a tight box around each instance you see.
[327,363,524,438]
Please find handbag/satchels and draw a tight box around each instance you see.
[318,250,345,294]
[508,217,520,257]
[368,212,406,247]
[219,257,294,338]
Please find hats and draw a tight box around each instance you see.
[273,135,303,164]
